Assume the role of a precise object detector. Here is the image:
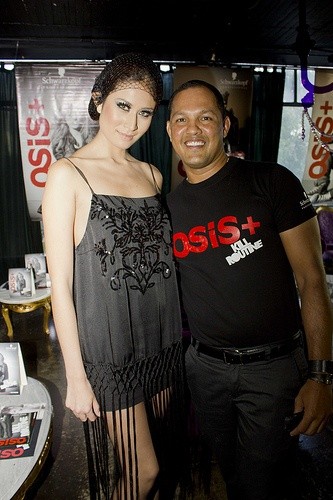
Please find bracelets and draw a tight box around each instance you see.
[306,373,333,385]
[307,359,333,374]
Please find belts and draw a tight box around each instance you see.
[190,338,305,366]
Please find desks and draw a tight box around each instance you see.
[0,377,56,500]
[0,273,51,342]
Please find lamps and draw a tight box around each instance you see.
[157,62,175,75]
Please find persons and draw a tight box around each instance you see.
[41,52,212,500]
[165,80,333,500]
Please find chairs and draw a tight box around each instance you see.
[315,206,333,274]
